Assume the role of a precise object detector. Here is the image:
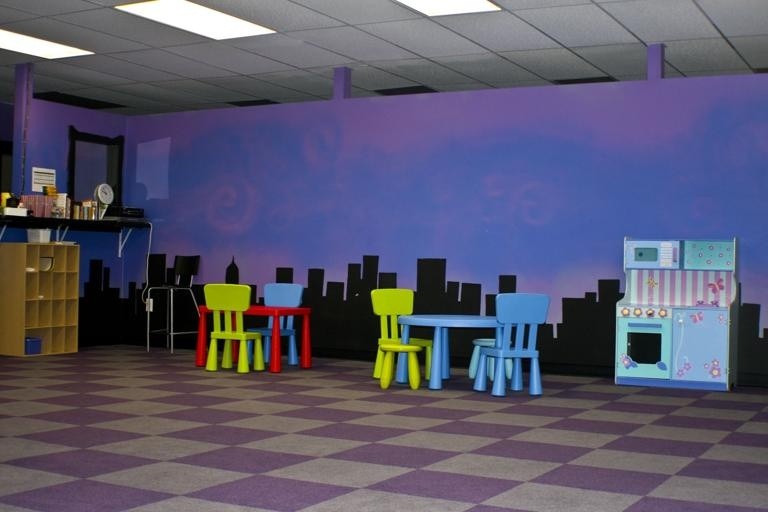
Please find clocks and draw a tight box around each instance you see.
[93,183,115,205]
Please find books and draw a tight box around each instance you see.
[21,193,108,220]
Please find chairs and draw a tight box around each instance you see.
[195,282,314,374]
[147,255,209,355]
[369,288,552,396]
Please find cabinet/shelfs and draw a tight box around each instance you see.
[0,243,81,358]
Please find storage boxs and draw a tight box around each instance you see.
[25,337,42,354]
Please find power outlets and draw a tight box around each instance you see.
[145,297,154,313]
